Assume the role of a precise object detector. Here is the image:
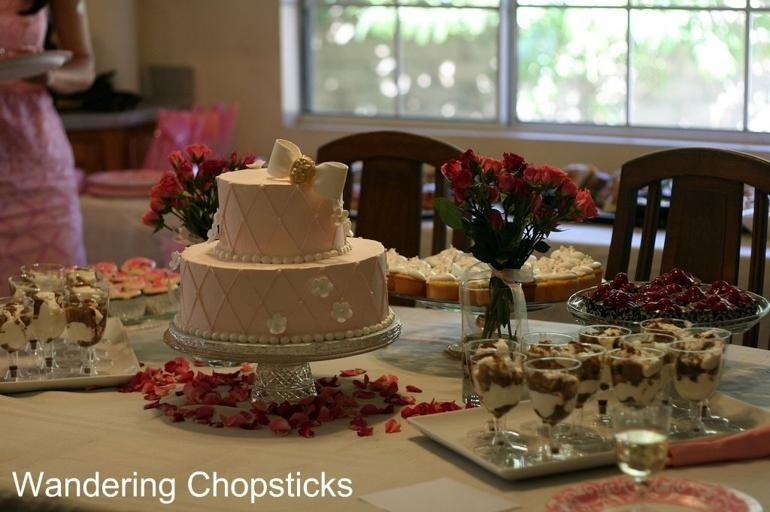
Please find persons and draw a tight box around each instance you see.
[0,0,96,293]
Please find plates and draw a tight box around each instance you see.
[401,386,769,485]
[519,473,767,511]
[0,314,143,395]
[2,47,75,83]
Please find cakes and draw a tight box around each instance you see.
[171,138,396,345]
[463,317,727,424]
[387,244,604,308]
[0,255,180,352]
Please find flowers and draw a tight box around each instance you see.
[142,143,258,245]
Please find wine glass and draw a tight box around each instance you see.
[462,315,742,469]
[611,402,674,511]
[0,261,112,381]
